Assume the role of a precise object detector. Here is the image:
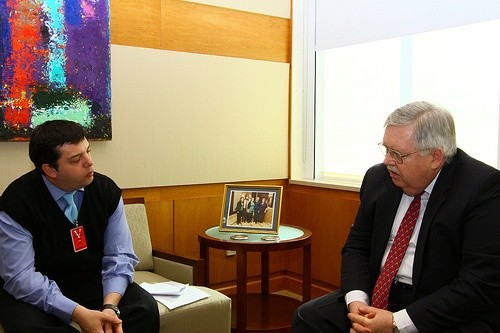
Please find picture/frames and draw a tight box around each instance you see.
[219,184,283,235]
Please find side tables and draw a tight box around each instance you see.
[198,225,312,333]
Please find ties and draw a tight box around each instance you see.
[371,190,426,311]
[63,190,79,225]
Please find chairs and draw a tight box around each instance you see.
[71,198,231,333]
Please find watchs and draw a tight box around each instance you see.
[392,313,400,333]
[99,304,120,318]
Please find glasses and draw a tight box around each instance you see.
[378,141,443,164]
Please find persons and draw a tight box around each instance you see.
[235,194,269,226]
[0,120,159,333]
[293,102,500,333]
